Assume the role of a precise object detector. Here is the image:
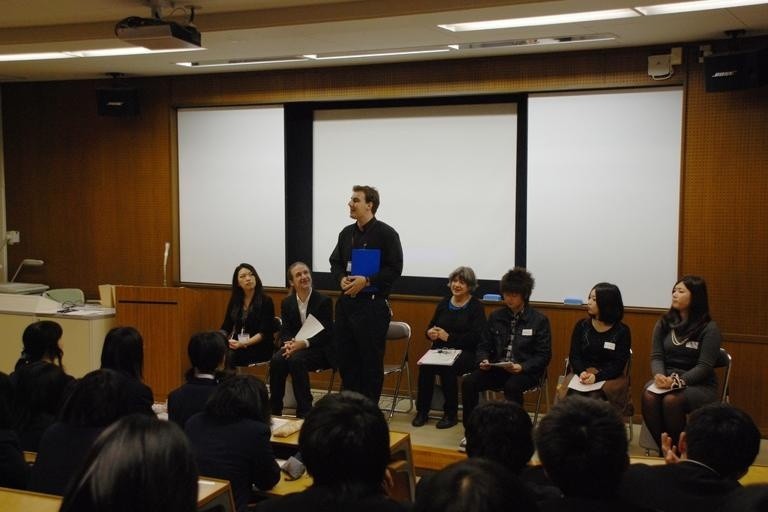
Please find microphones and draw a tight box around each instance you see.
[163,241,171,287]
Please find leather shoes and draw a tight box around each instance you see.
[413,411,427,426]
[436,411,457,429]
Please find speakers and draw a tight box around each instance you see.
[704,47,767,93]
[95,85,141,116]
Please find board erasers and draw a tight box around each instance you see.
[483,294,501,302]
[564,298,582,305]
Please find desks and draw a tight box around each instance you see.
[0,293,118,380]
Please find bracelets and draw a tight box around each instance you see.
[365,276,371,287]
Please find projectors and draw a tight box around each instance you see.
[115,15,201,51]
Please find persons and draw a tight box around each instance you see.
[266,261,333,418]
[458,268,553,455]
[256,391,418,512]
[411,265,487,430]
[558,282,633,419]
[414,396,768,512]
[185,262,279,382]
[1,321,158,496]
[167,332,281,512]
[327,185,404,406]
[58,412,199,512]
[640,273,723,457]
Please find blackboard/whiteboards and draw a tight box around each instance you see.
[168,86,690,315]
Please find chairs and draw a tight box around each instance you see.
[41,287,85,305]
